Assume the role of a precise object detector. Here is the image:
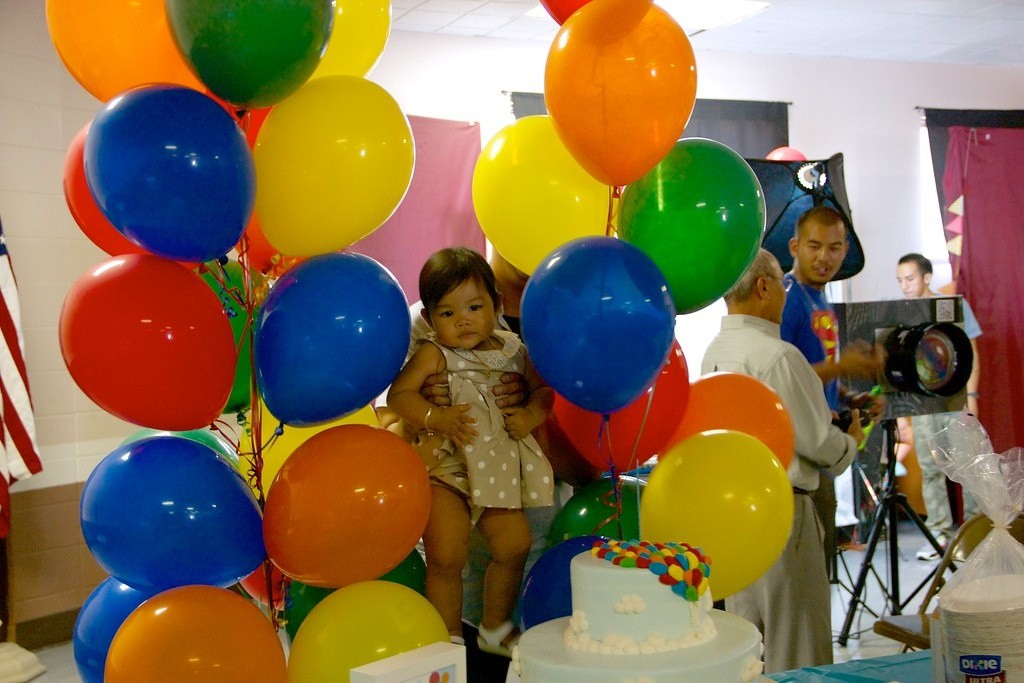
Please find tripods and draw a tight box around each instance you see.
[840,421,959,648]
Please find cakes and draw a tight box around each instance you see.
[512,538,766,682]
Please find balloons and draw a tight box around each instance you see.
[47,0,454,683]
[471,0,807,596]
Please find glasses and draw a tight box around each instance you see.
[754,272,793,292]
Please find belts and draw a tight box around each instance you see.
[793,486,812,497]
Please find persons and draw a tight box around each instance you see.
[896,253,985,561]
[701,244,866,673]
[388,246,556,660]
[374,243,576,683]
[779,207,889,584]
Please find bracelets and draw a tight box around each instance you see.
[967,390,979,399]
[423,406,437,436]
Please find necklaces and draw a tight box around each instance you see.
[470,337,497,378]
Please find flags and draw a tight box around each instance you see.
[0,221,43,541]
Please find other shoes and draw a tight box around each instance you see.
[951,545,967,562]
[916,534,950,561]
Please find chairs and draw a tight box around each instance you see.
[872,515,1024,654]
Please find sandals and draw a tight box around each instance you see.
[477,619,522,658]
[449,635,465,646]
